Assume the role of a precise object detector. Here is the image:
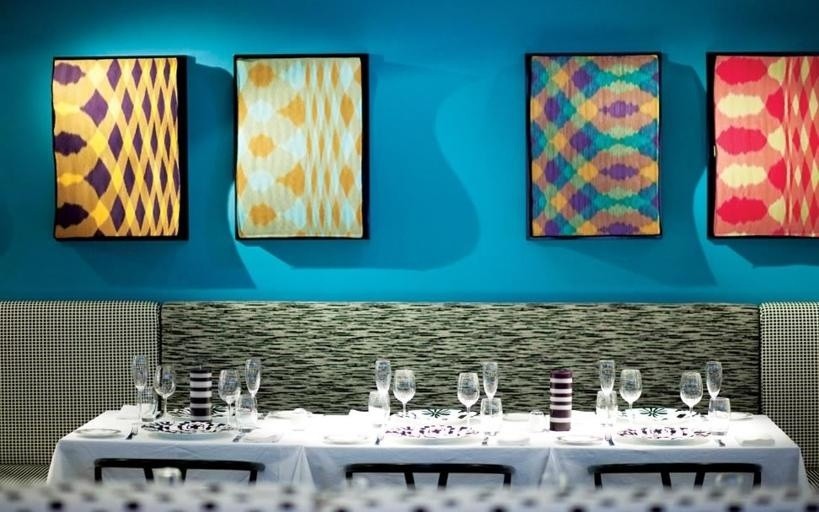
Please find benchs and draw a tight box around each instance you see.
[161,302,762,414]
[762,303,818,468]
[0,301,159,484]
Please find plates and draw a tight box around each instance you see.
[384,408,480,442]
[609,407,712,444]
[75,428,120,437]
[142,419,241,440]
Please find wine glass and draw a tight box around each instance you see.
[130,356,176,425]
[368,359,416,434]
[457,361,502,433]
[679,360,730,432]
[594,360,643,448]
[218,359,262,431]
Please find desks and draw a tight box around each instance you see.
[54,401,802,489]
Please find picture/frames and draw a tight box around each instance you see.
[525,52,663,238]
[235,52,369,241]
[54,55,190,243]
[707,50,819,238]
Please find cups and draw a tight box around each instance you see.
[189,369,212,421]
[548,367,574,430]
